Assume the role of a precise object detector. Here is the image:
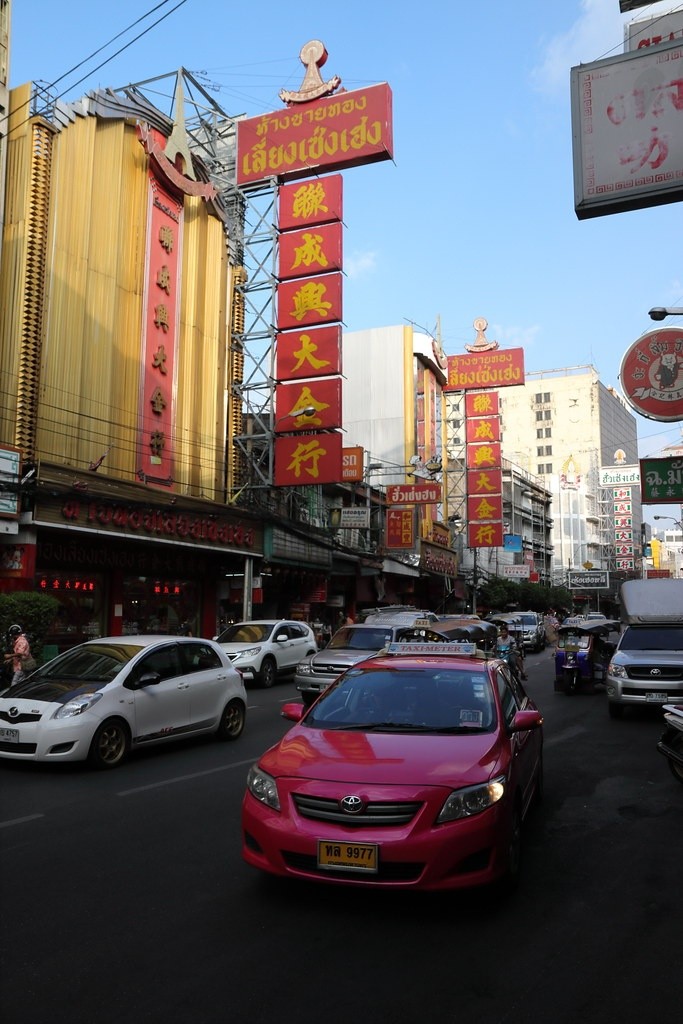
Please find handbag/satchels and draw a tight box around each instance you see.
[21,658,37,671]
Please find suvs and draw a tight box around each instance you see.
[210,619,318,690]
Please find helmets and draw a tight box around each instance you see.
[8,625,22,636]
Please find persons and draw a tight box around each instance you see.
[323,618,332,648]
[495,628,517,673]
[493,625,529,682]
[336,611,346,627]
[4,625,33,686]
[344,613,354,626]
[176,615,192,637]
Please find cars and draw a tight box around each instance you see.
[235,641,547,903]
[1,634,249,770]
[296,625,452,715]
[363,577,683,723]
